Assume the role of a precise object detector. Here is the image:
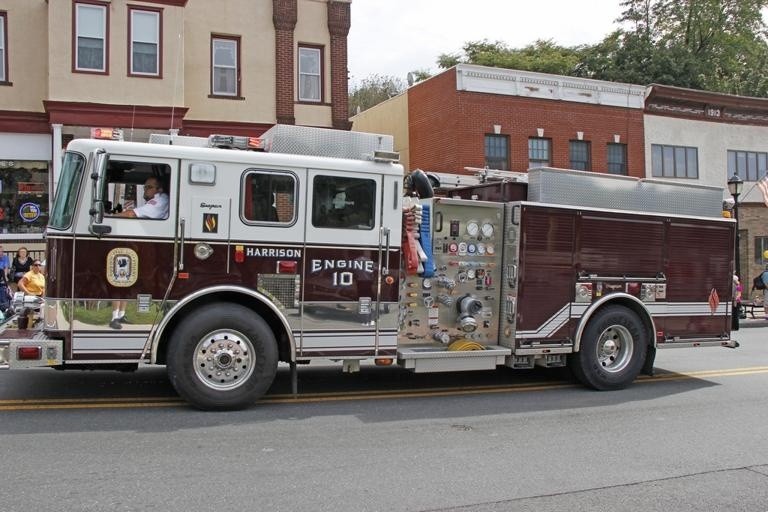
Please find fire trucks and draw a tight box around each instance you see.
[40,123,737,409]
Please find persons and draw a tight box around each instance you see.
[110,177,169,220]
[760,263,768,321]
[349,252,376,328]
[0,246,47,301]
[109,299,133,329]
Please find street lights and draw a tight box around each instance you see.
[726,172,742,283]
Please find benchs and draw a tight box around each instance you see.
[744,302,767,320]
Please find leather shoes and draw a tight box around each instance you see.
[119,315,134,324]
[109,318,122,329]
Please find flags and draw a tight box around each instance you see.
[756,174,768,207]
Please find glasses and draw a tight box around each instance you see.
[144,184,159,188]
[33,264,41,266]
